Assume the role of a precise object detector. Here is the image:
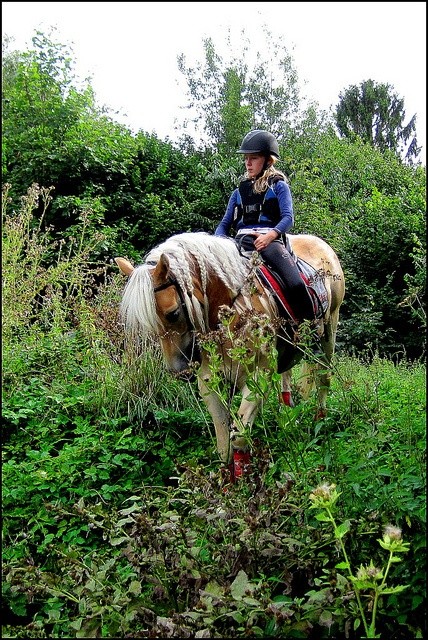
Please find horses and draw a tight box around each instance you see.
[114,230,345,495]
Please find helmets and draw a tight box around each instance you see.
[236,129,279,157]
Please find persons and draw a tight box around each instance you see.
[215,130,324,364]
